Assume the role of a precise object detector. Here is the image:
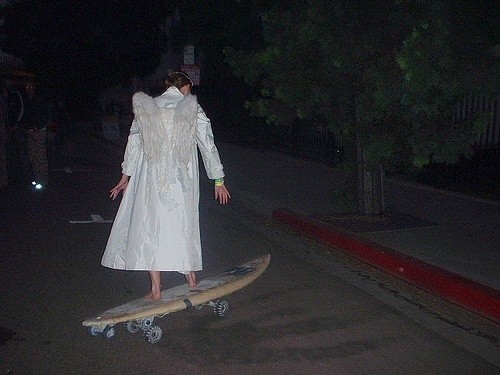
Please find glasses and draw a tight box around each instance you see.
[24,85,36,89]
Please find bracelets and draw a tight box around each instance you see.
[214,178,225,186]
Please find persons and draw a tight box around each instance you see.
[9,80,62,188]
[110,72,232,298]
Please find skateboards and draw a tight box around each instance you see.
[81,253,272,343]
